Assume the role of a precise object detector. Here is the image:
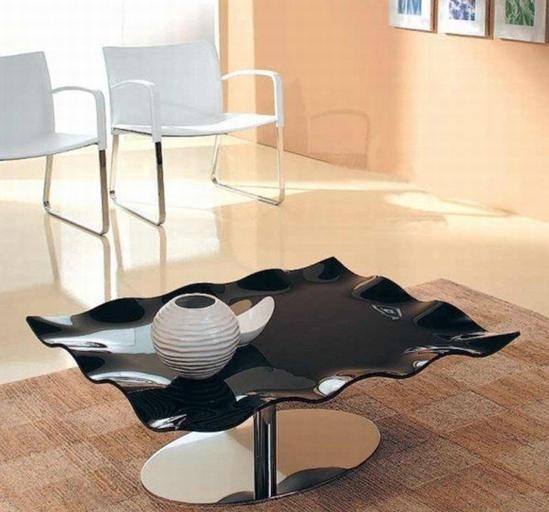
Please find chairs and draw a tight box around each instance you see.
[102,41,287,226]
[1,51,109,235]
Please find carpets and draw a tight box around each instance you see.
[0,277,546,511]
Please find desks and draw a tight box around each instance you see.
[23,255,519,511]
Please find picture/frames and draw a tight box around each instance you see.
[387,0,549,45]
